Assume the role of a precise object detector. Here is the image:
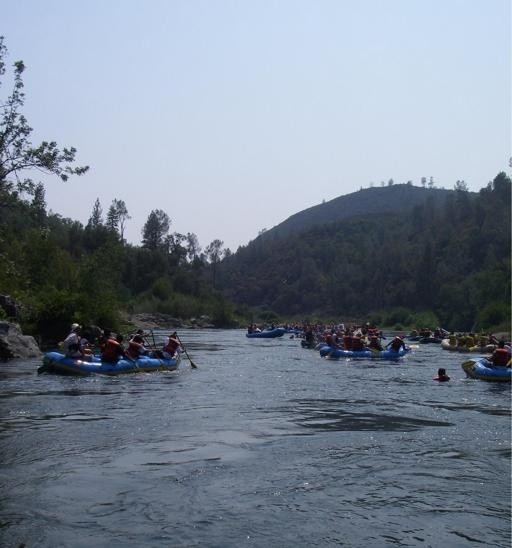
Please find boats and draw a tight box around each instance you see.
[289,327,411,357]
[42,351,181,373]
[461,357,512,381]
[247,327,285,338]
[407,333,497,353]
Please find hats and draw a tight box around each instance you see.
[70,323,82,332]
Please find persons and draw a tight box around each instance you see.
[246,317,512,381]
[62,323,180,362]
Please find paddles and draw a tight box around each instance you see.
[366,347,379,355]
[176,333,197,369]
[406,345,419,349]
[38,352,75,374]
[506,358,511,367]
